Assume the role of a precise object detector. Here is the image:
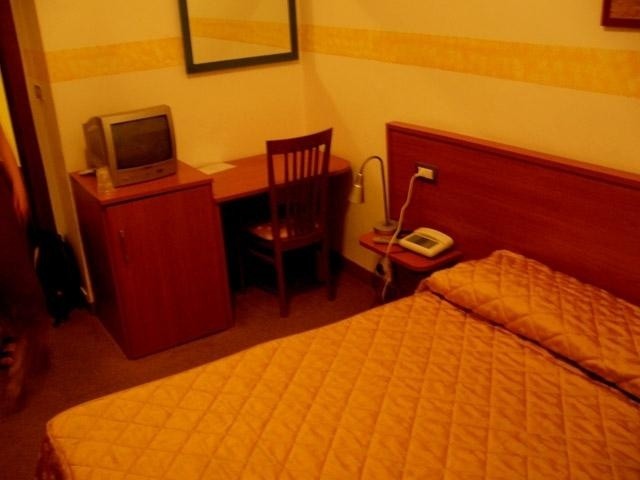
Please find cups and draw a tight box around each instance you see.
[95,167,115,195]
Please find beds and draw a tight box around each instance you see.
[39,250,640,480]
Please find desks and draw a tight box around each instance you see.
[359,224,463,277]
[191,143,352,267]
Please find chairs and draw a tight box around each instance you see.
[241,128,340,316]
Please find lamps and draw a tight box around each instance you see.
[349,154,401,237]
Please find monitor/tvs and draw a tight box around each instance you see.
[83,104,179,188]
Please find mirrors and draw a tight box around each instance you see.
[179,0,300,81]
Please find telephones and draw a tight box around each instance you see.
[398,227,454,258]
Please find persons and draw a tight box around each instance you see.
[2,128,60,385]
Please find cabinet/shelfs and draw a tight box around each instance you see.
[71,158,232,363]
